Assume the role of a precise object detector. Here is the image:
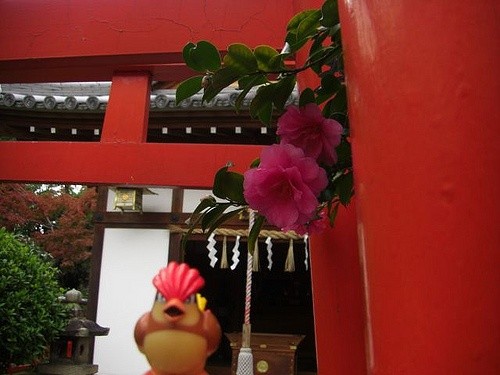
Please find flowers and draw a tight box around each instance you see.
[175,0,354,254]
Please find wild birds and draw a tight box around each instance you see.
[134,262,221,375]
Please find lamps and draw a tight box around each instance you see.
[109,186,158,216]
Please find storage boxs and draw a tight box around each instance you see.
[205,333,307,375]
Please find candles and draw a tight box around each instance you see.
[66,340,72,359]
